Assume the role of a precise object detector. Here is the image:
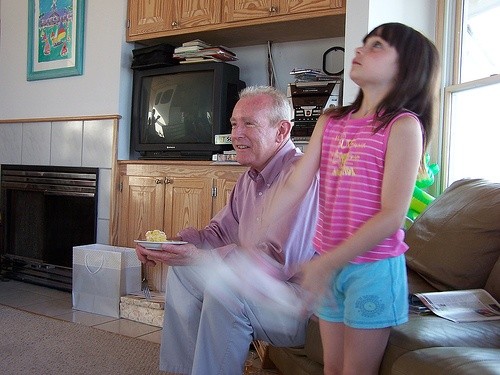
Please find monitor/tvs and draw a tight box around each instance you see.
[131,62,246,162]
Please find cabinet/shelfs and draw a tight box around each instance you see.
[128,0,346,50]
[117,160,242,291]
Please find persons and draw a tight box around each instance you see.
[235,23,440,375]
[135,84,321,375]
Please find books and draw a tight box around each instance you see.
[172,40,238,66]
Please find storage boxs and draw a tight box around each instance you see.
[120,296,163,328]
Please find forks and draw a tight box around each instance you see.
[141,262,152,300]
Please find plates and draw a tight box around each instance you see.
[135,240,189,251]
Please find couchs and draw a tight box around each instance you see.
[268,179,500,375]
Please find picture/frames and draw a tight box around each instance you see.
[27,0,84,81]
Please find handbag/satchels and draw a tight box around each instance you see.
[71,243,143,318]
